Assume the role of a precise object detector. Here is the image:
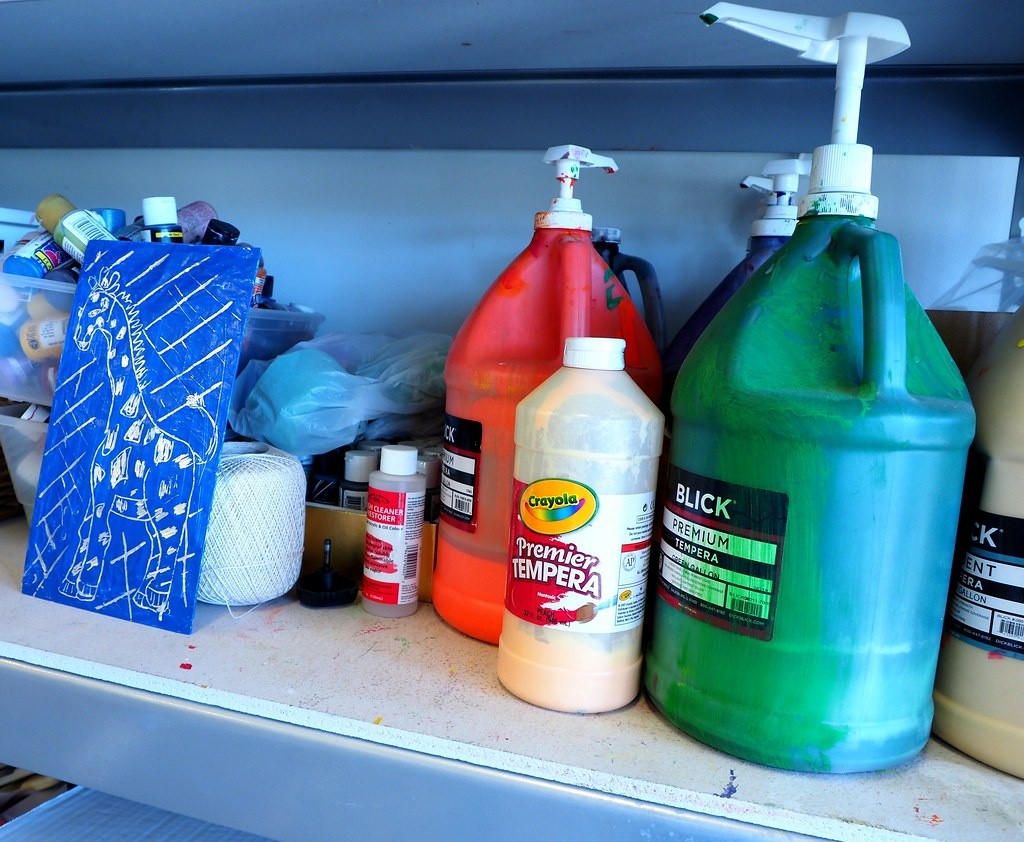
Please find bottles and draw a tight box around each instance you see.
[497,337,665,713]
[339,438,443,618]
[0,193,328,396]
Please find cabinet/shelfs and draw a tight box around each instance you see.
[0,0,1024,842]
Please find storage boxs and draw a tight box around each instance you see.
[0,403,48,507]
[0,271,325,407]
